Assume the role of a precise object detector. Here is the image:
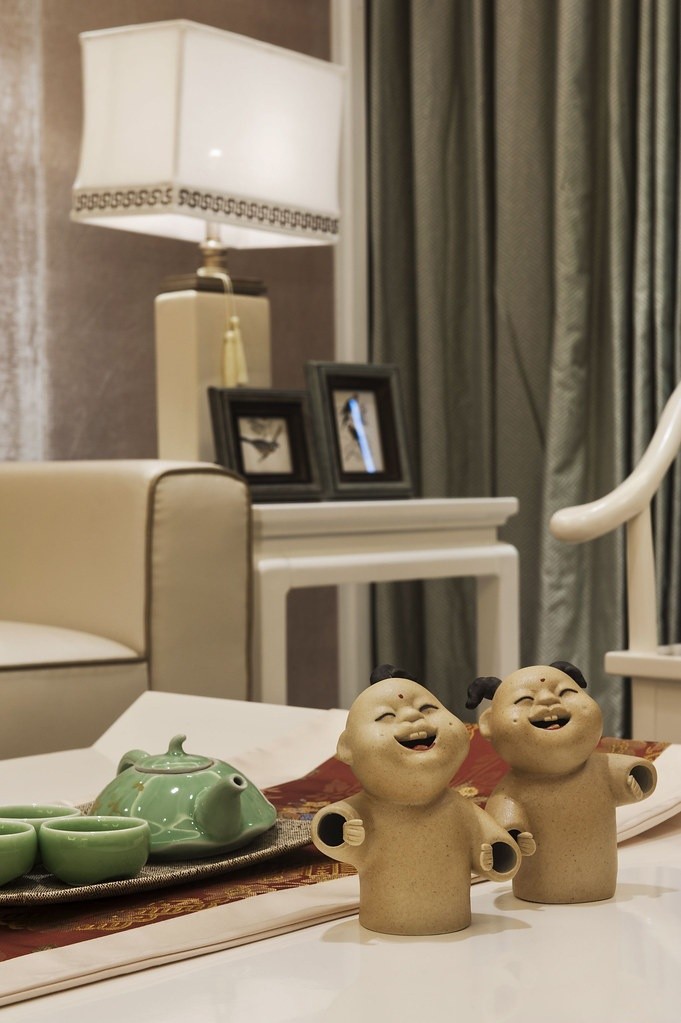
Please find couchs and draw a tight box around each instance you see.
[0,457,252,767]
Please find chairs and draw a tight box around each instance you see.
[546,377,680,747]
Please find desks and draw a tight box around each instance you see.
[0,726,677,1020]
[242,496,520,720]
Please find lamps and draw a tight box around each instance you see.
[67,19,349,464]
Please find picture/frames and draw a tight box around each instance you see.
[207,387,324,504]
[302,360,417,501]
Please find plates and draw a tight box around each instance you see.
[1,819,313,907]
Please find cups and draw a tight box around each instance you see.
[38,814,152,888]
[0,822,38,890]
[1,805,83,842]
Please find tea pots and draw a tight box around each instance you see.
[88,733,278,860]
